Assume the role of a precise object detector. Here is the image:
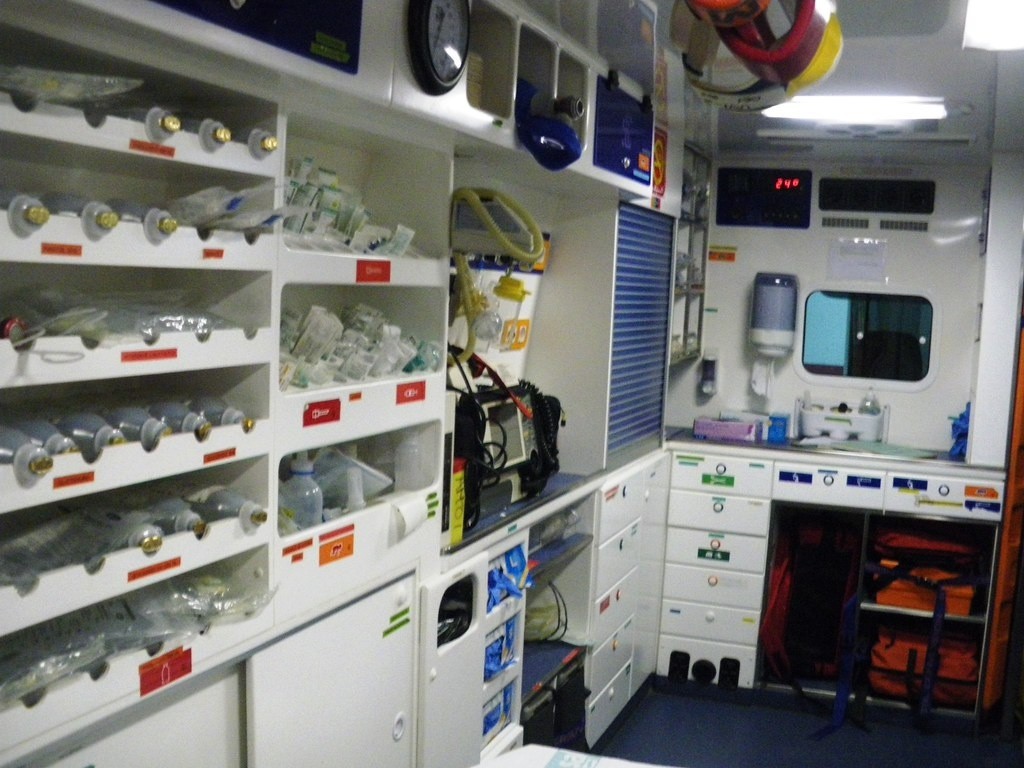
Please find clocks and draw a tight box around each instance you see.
[407,0,471,95]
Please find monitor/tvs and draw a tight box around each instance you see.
[477,393,539,474]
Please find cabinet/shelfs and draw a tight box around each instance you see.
[0,0,1024,768]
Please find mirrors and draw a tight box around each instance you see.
[792,282,941,392]
